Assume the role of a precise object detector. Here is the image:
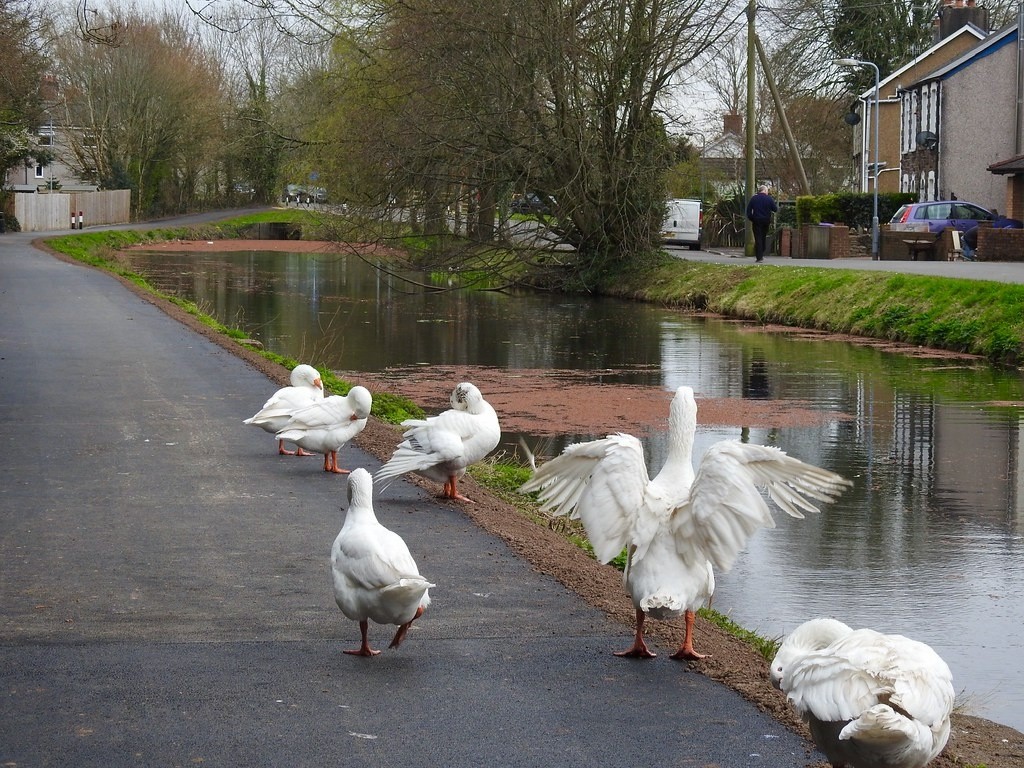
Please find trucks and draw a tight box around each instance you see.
[657,198,703,251]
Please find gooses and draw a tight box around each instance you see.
[243,364,372,474]
[373,381,501,503]
[331,466,437,657]
[516,385,855,659]
[770,618,956,768]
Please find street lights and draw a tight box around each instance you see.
[44,110,53,194]
[686,131,706,203]
[832,58,880,260]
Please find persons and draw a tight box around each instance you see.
[746,184,778,263]
[960,223,980,262]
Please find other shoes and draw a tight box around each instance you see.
[755,256,764,263]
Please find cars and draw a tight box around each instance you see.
[887,199,1024,241]
[282,184,301,202]
[511,193,559,216]
[297,185,328,203]
